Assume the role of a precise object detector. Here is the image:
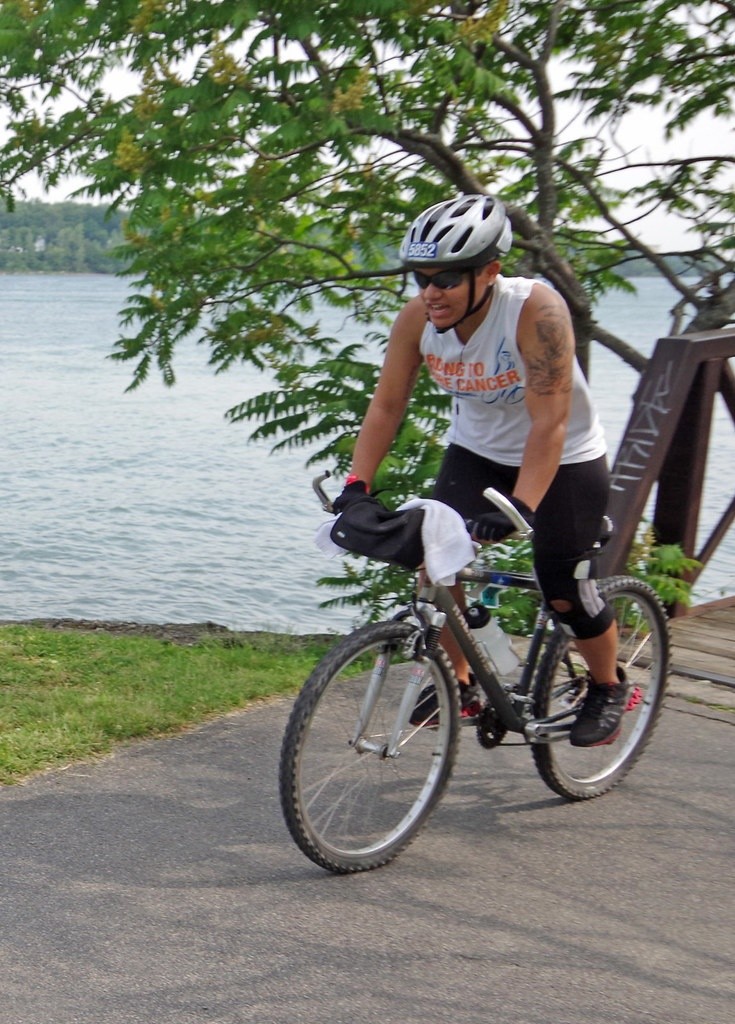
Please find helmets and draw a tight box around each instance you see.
[396,194,514,270]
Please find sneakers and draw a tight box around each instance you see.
[409,672,482,727]
[570,666,629,747]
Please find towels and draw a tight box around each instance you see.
[393,499,476,587]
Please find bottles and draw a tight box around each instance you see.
[468,601,520,675]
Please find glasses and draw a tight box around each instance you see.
[413,269,471,289]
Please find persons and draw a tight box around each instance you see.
[331,192,630,747]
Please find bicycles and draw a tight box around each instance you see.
[274,471,675,878]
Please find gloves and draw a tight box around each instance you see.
[331,474,371,516]
[465,496,537,543]
